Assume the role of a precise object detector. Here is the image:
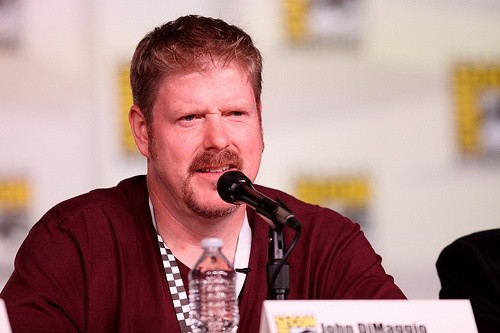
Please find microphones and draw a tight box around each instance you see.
[236,268,251,273]
[217,171,300,229]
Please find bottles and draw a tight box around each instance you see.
[188,238,240,333]
